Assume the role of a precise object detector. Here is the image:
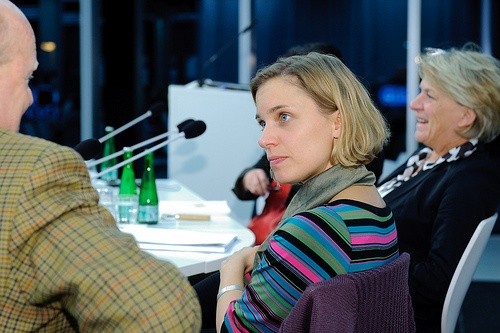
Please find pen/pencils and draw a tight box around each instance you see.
[175,215,210,221]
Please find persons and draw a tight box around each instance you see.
[190,51,500,333]
[0,0,202,333]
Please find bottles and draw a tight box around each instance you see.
[101,126,118,184]
[138,149,159,224]
[118,147,137,223]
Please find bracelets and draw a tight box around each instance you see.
[216,285,247,300]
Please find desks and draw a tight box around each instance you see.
[115,179,256,278]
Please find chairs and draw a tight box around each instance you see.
[279,216,498,333]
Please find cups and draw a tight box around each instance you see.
[115,194,137,223]
[93,185,116,222]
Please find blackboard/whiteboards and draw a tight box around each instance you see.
[168,85,263,225]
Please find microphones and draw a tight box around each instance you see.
[199,19,260,88]
[74,102,206,179]
